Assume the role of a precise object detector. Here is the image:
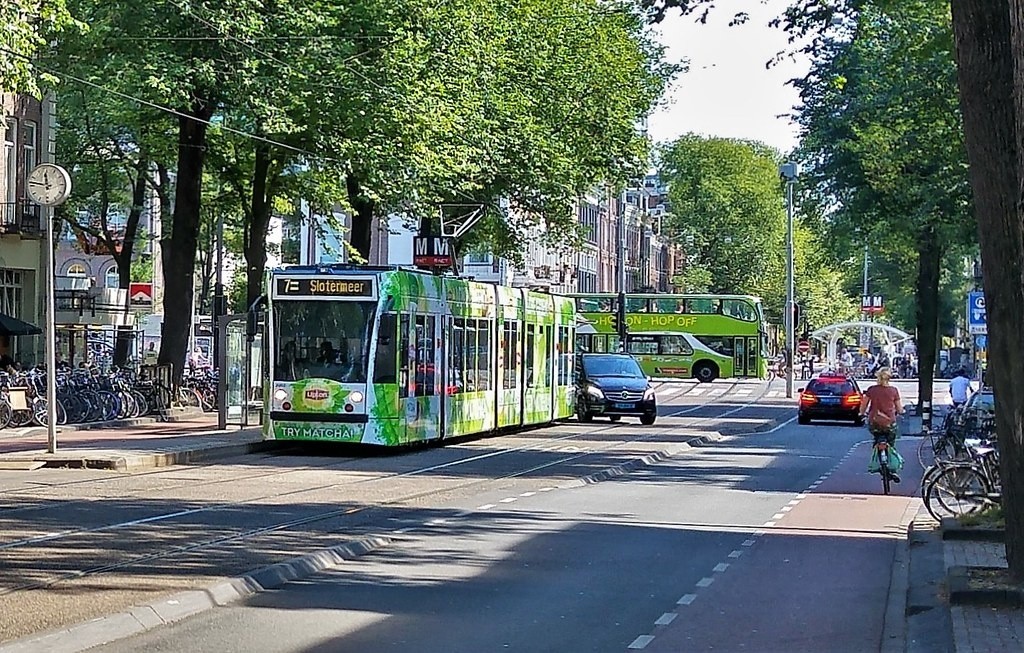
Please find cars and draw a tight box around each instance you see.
[797,371,868,427]
[573,351,658,425]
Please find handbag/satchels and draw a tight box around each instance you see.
[868,442,905,473]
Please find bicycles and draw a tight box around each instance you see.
[858,410,906,495]
[0,337,221,429]
[766,360,814,382]
[916,389,1003,525]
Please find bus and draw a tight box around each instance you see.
[243,196,581,456]
[562,289,772,385]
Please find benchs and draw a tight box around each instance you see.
[242,401,266,426]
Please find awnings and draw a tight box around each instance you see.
[0,313,42,338]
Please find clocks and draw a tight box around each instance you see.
[26,165,66,206]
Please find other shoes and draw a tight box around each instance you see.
[893,474,901,483]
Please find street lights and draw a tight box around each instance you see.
[778,161,799,399]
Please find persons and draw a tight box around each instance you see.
[774,348,787,373]
[189,359,196,377]
[283,342,296,371]
[144,342,158,365]
[56,352,69,370]
[839,348,890,377]
[808,356,815,378]
[949,369,974,408]
[318,341,335,364]
[859,367,906,484]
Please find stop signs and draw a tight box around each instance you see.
[798,341,809,353]
